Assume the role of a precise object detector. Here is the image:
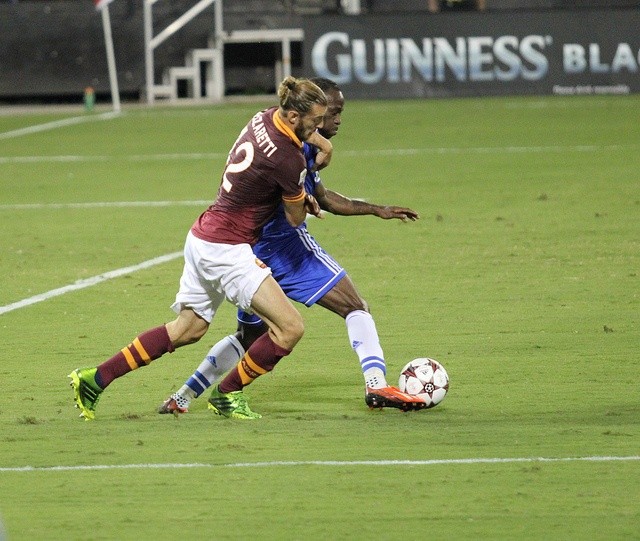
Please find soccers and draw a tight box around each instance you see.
[398,357,450,409]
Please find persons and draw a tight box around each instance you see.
[65,75,333,419]
[157,78,427,412]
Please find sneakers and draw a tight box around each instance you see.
[67,368,106,418]
[365,385,426,412]
[208,385,260,417]
[158,399,189,413]
